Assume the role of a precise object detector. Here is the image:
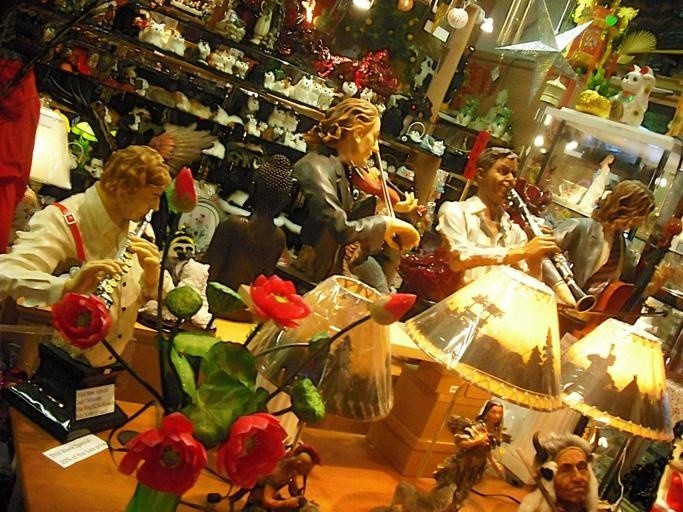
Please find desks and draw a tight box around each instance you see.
[1,293,539,512]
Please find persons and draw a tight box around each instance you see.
[434,401,512,512]
[0,145,175,367]
[438,148,562,287]
[518,430,601,512]
[555,180,674,313]
[579,155,614,208]
[203,155,293,293]
[244,446,322,512]
[285,98,418,280]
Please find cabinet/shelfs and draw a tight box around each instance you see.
[0,0,683,308]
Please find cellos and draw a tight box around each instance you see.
[572,215,683,343]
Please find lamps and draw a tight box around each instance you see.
[248,276,398,448]
[560,320,676,498]
[404,266,565,485]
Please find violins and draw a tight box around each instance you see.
[344,161,427,220]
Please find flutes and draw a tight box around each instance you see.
[92,208,153,311]
